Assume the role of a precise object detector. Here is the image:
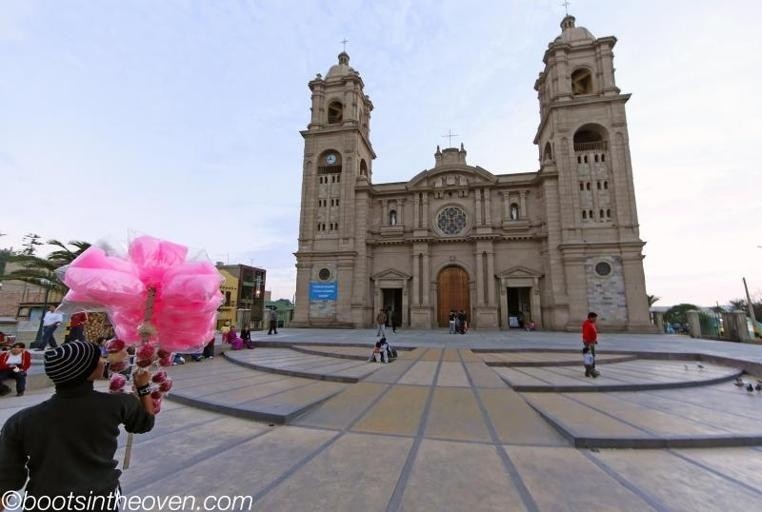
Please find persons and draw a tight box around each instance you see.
[176,336,215,364]
[0,304,135,398]
[0,341,155,512]
[267,307,278,335]
[582,312,601,376]
[448,309,466,335]
[227,324,244,350]
[662,320,688,335]
[582,343,596,378]
[517,311,525,332]
[220,320,231,344]
[240,324,255,349]
[369,305,396,364]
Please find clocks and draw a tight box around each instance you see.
[326,154,337,165]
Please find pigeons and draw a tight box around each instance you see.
[697,364,704,368]
[732,375,762,392]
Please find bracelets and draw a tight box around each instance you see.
[136,382,152,397]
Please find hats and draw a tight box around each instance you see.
[44,342,101,384]
